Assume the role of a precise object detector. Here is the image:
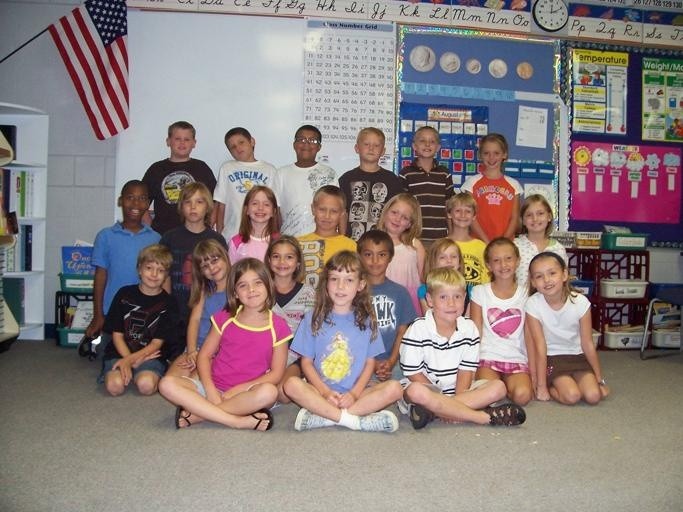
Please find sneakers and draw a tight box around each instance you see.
[77,336,94,357]
[359,409,399,433]
[295,408,325,432]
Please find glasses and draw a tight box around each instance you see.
[198,255,223,273]
[294,137,321,145]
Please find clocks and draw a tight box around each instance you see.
[532,0,571,32]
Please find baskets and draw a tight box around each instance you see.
[552,232,602,249]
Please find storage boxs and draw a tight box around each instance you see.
[60,328,93,348]
[58,274,96,293]
[555,228,680,349]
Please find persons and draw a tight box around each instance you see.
[525,252,608,405]
[78,180,161,358]
[511,195,569,284]
[141,121,532,431]
[101,244,172,394]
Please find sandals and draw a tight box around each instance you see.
[407,403,439,431]
[483,403,526,426]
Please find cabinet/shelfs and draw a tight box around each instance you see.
[1,101,51,344]
[54,289,96,345]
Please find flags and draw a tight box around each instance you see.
[46,1,130,141]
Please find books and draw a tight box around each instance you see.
[605,300,682,332]
[0,124,36,327]
[59,239,95,330]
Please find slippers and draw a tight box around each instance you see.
[175,406,191,429]
[250,408,274,430]
